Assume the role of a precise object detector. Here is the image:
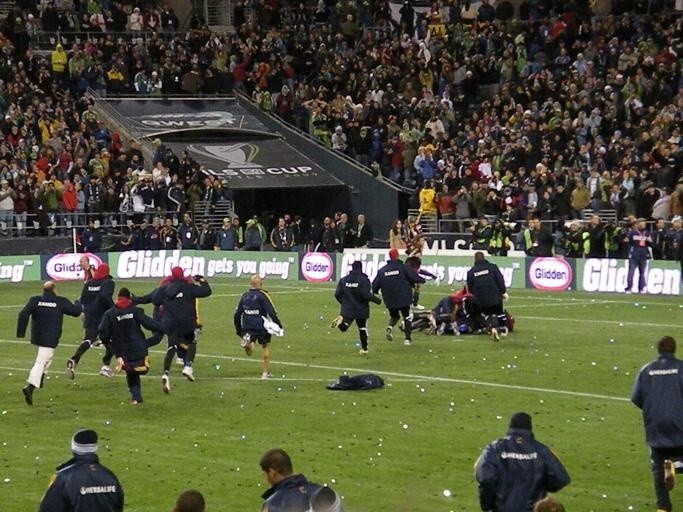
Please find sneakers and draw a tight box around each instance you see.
[663,459,675,492]
[241,332,251,348]
[385,303,503,347]
[115,356,196,405]
[99,365,114,378]
[359,348,369,356]
[23,385,34,406]
[261,371,274,378]
[329,315,344,329]
[66,359,76,380]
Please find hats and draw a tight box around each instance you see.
[636,218,647,224]
[223,218,256,225]
[151,138,161,145]
[71,429,98,456]
[509,412,532,431]
[352,261,362,271]
[389,249,399,258]
[171,266,184,278]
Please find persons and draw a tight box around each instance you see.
[1,0,683,267]
[631,333,683,512]
[64,256,212,406]
[233,273,283,379]
[471,409,572,512]
[38,426,125,510]
[534,495,566,512]
[329,260,382,356]
[173,488,205,511]
[15,279,82,406]
[623,217,654,292]
[257,447,325,511]
[371,248,515,345]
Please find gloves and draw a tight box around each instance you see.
[193,275,204,281]
